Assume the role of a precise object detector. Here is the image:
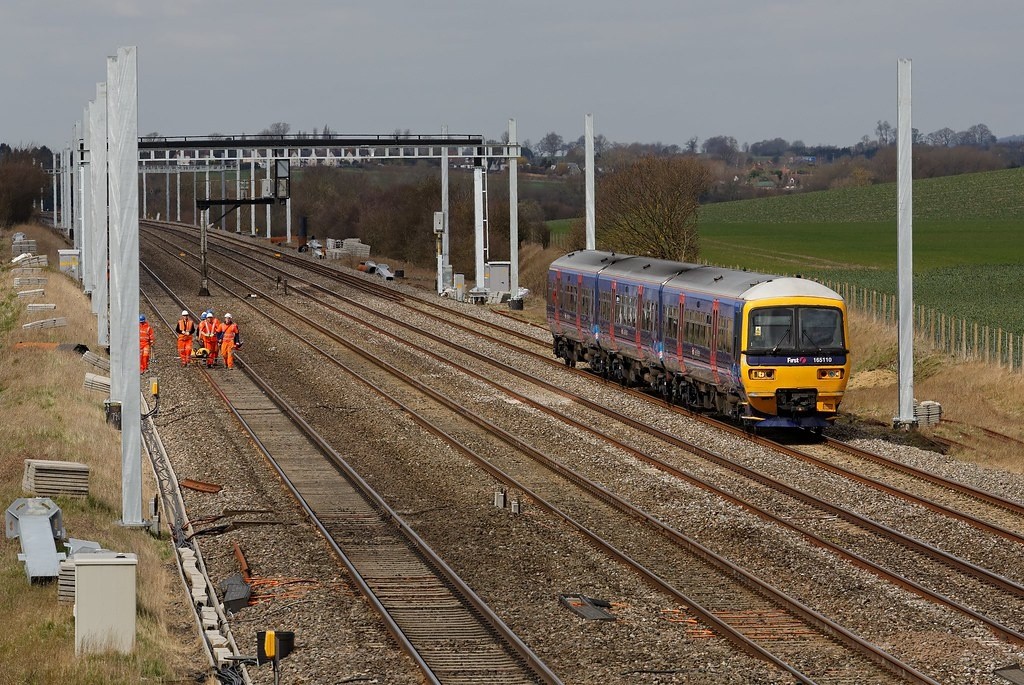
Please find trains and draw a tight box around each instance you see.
[547,249,852,440]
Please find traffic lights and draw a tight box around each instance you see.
[278,632,296,662]
[257,630,276,668]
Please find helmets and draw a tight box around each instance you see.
[206,309,212,313]
[139,315,146,322]
[181,310,188,315]
[224,313,232,318]
[206,313,213,318]
[201,312,207,319]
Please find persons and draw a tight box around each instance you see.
[195,309,243,370]
[139,315,155,375]
[175,310,195,368]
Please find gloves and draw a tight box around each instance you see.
[210,333,214,337]
[182,330,186,334]
[236,342,239,345]
[204,334,210,337]
[184,332,190,336]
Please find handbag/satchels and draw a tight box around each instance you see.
[218,332,224,339]
